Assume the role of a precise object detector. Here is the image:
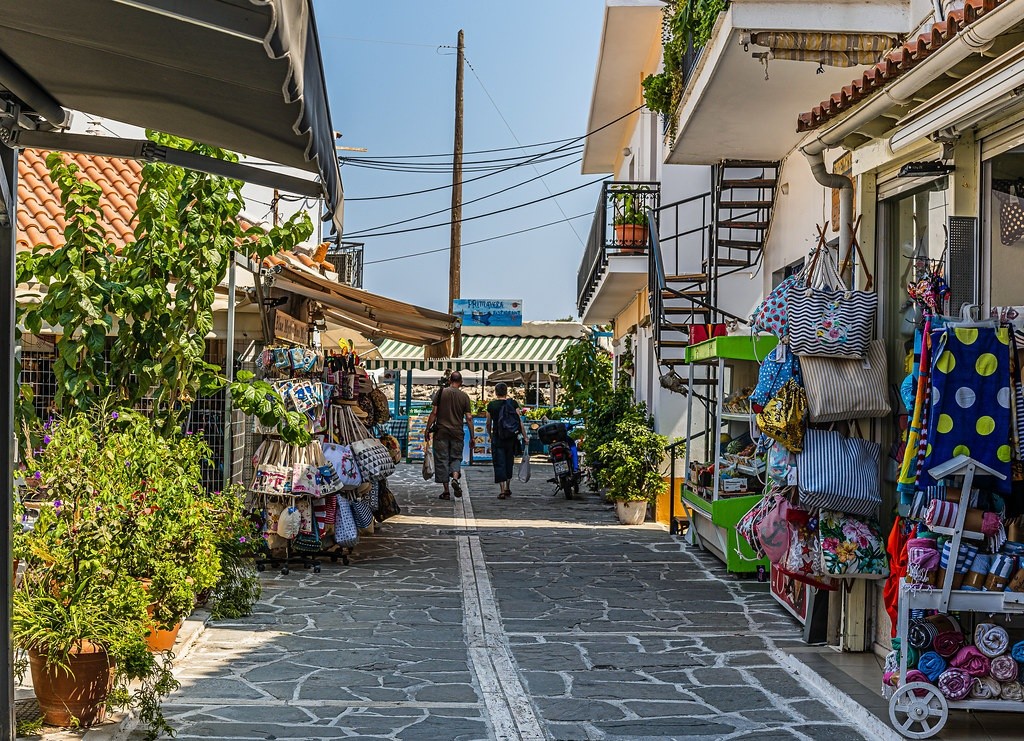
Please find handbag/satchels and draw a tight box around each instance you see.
[422,439,435,480]
[734,342,808,570]
[247,353,402,561]
[799,337,891,423]
[775,506,839,592]
[429,416,438,433]
[796,419,883,517]
[518,444,531,483]
[746,247,817,341]
[788,250,878,359]
[818,507,890,579]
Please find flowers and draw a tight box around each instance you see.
[474,400,488,412]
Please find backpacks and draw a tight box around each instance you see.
[498,398,521,440]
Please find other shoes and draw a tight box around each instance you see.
[439,491,450,500]
[504,489,512,496]
[497,493,505,499]
[451,479,462,498]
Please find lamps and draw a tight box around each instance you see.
[896,161,956,177]
[623,147,632,156]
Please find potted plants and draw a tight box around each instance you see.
[569,387,688,525]
[608,184,658,253]
[11,515,186,741]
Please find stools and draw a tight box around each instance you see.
[675,515,692,535]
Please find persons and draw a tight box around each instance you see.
[425,370,475,500]
[487,383,529,499]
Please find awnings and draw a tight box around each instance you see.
[275,262,462,360]
[376,336,582,372]
[0,0,347,245]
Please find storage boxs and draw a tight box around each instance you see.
[687,403,767,491]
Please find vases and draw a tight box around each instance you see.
[478,411,486,418]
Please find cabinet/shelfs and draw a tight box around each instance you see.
[684,335,779,577]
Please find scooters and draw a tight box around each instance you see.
[538,419,584,501]
